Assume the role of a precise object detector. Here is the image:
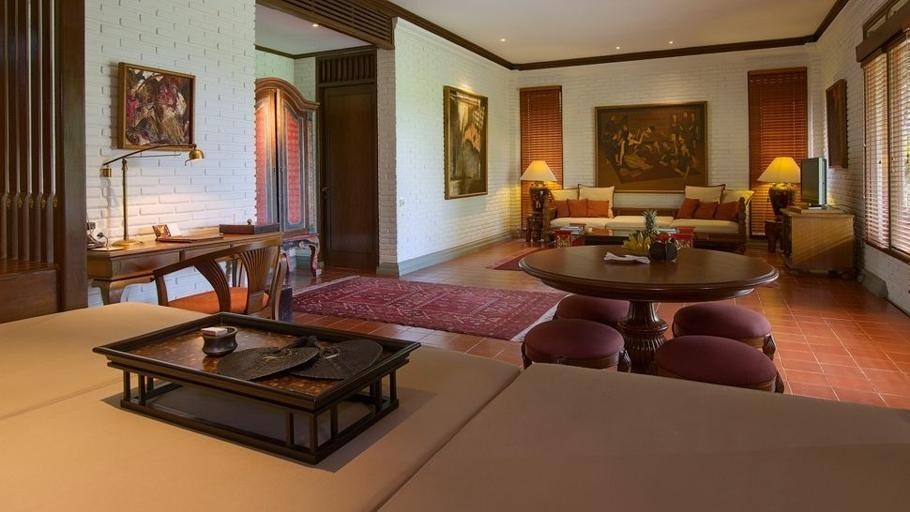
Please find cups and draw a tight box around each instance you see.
[201,326,237,356]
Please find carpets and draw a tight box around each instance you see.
[484,246,555,272]
[292,274,569,342]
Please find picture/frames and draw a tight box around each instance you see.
[442,83,489,201]
[824,75,850,171]
[117,61,197,154]
[592,100,710,196]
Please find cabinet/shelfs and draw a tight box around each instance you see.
[255,75,322,286]
[778,206,857,277]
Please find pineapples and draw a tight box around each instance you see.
[641,208,660,242]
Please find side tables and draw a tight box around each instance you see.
[766,219,783,251]
[525,206,559,243]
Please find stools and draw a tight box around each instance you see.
[671,299,776,361]
[519,317,632,373]
[651,334,786,394]
[553,295,630,340]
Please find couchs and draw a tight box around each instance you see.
[539,207,748,256]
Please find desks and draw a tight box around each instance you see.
[88,230,283,307]
[517,244,782,374]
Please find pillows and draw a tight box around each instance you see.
[553,198,571,219]
[586,199,611,218]
[694,201,720,219]
[565,198,589,218]
[683,184,725,203]
[674,196,702,220]
[718,187,756,205]
[713,201,741,223]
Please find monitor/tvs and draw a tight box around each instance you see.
[800,158,826,209]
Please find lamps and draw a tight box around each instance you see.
[519,160,560,212]
[101,143,206,250]
[756,156,801,222]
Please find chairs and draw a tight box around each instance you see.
[152,237,288,322]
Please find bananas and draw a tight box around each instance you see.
[623,232,651,254]
